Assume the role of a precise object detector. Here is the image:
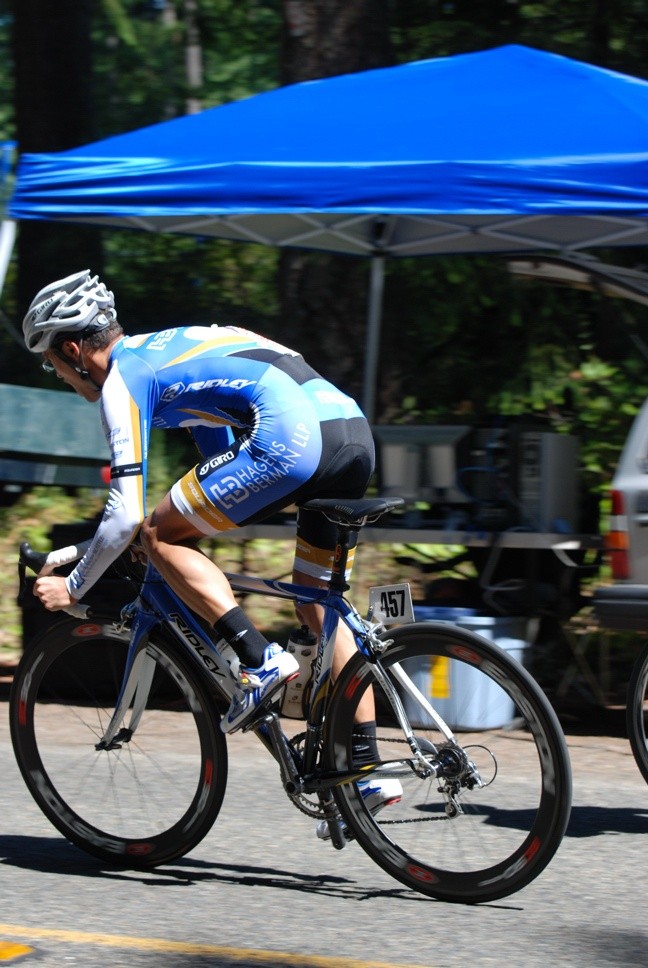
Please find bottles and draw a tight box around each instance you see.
[278,625,318,719]
[211,632,241,679]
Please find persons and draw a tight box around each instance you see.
[395,440,558,620]
[22,268,404,840]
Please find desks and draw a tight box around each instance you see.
[209,522,601,723]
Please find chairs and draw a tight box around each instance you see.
[549,543,605,713]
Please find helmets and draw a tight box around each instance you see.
[22,269,118,352]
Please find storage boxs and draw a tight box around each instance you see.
[394,609,534,734]
[374,424,467,493]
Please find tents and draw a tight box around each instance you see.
[0,46,648,483]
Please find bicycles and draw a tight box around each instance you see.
[8,494,574,905]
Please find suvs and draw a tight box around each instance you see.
[592,393,647,779]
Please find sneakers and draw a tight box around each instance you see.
[317,774,402,839]
[220,644,299,732]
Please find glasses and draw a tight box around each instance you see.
[42,349,58,371]
